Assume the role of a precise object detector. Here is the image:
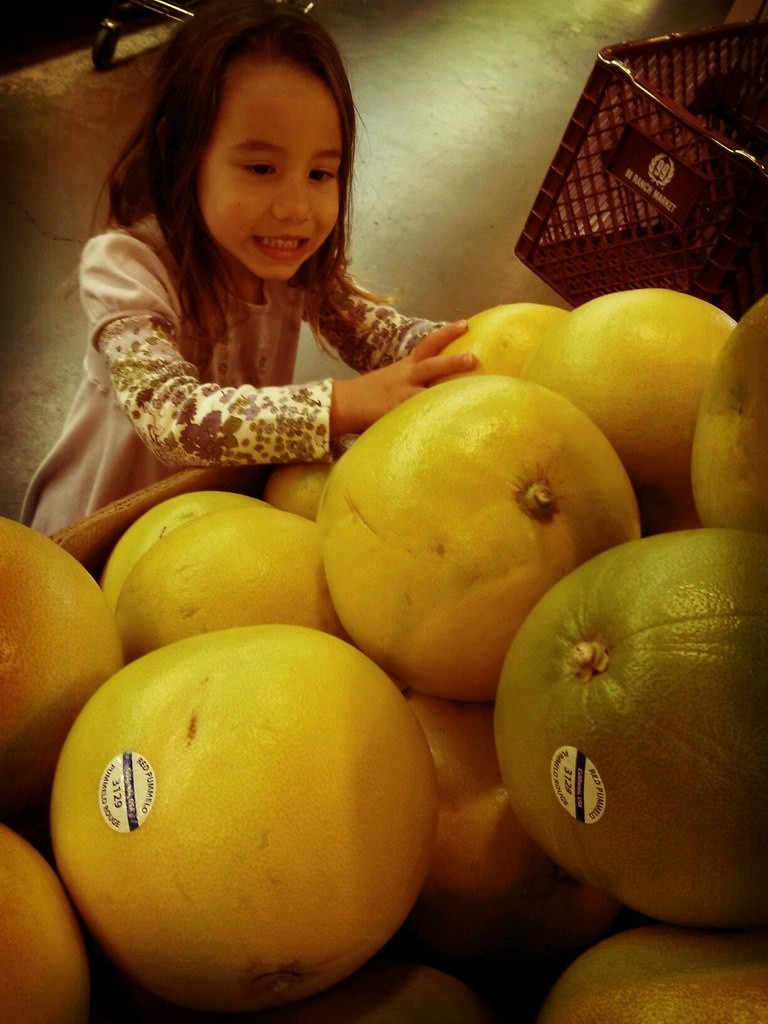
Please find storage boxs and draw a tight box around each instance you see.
[513,15,768,319]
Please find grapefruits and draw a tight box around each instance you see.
[0,287,768,1024]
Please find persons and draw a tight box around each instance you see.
[21,0,479,537]
